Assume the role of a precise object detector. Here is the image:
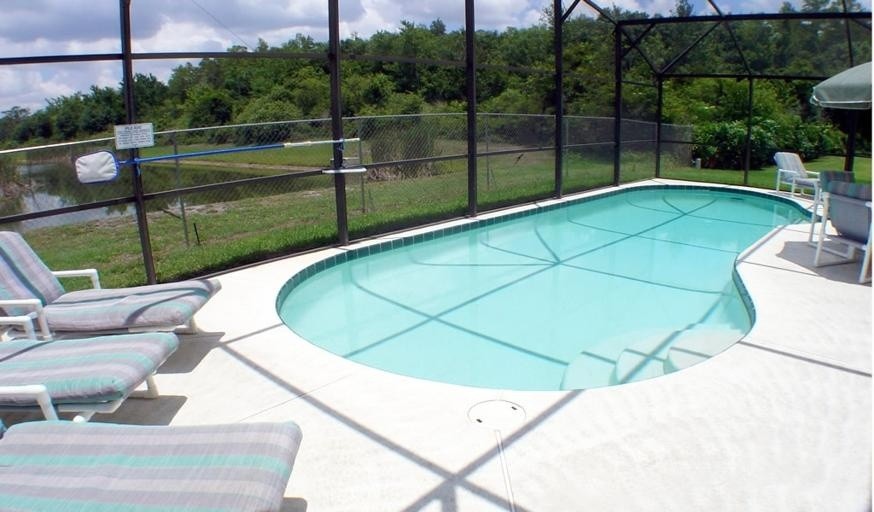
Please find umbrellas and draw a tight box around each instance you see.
[806,59,872,109]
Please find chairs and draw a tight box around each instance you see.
[773,151,872,284]
